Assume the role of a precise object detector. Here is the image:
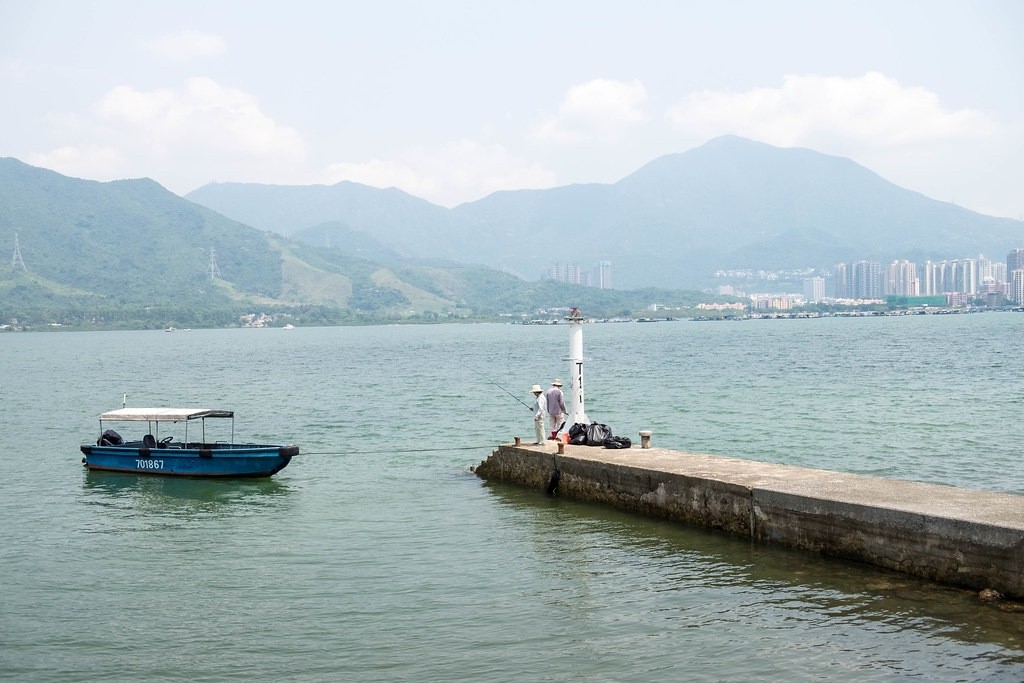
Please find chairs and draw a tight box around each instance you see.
[143,435,166,449]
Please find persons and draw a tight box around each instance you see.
[546,379,569,440]
[529,385,547,445]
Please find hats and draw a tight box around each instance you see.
[529,385,543,393]
[550,379,562,387]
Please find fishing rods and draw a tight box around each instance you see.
[457,358,534,412]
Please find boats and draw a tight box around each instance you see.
[79,392,300,479]
